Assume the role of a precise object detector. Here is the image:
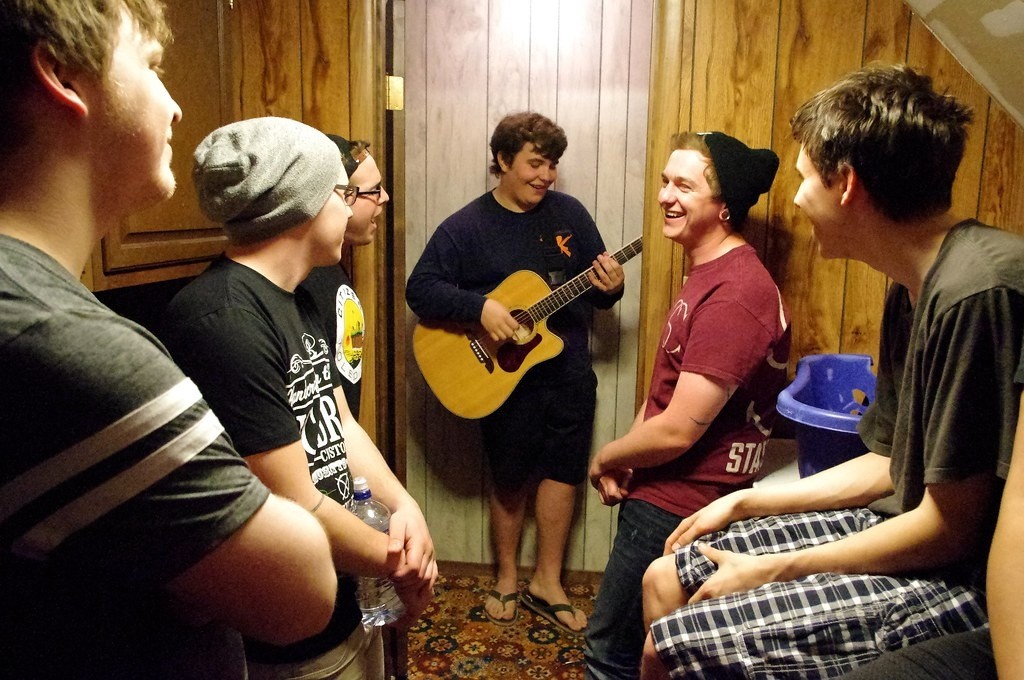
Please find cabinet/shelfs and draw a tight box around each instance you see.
[78,0,236,294]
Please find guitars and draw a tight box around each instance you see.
[411,236,644,420]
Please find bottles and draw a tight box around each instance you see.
[348,476,407,628]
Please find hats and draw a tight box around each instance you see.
[191,117,342,247]
[695,132,780,233]
[325,132,370,179]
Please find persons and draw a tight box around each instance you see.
[0,0,339,680]
[404,112,625,636]
[580,129,793,680]
[639,60,1024,680]
[152,115,438,680]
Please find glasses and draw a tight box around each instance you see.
[334,184,360,206]
[358,182,382,200]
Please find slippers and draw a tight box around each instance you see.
[519,586,587,636]
[483,589,519,626]
